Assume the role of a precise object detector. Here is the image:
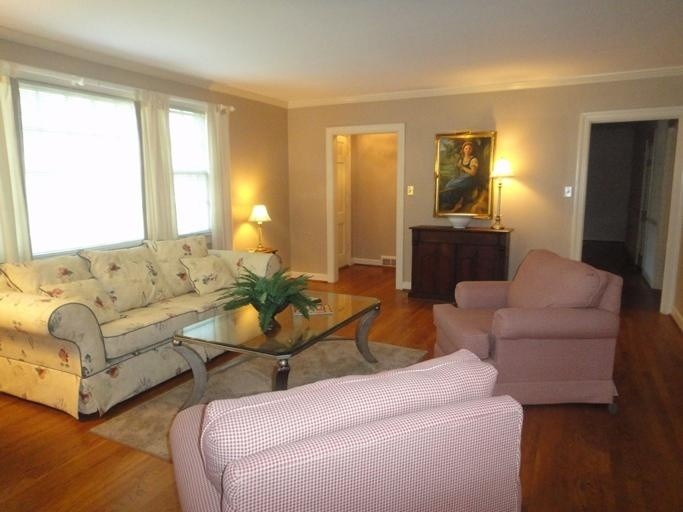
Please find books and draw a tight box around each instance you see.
[292,301,333,315]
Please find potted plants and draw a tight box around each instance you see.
[217,260,321,329]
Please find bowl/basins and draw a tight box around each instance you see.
[448,216,473,229]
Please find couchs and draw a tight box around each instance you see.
[433,248,623,406]
[0,234,283,421]
[166,348,524,512]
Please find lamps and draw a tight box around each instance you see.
[489,158,516,230]
[247,204,272,251]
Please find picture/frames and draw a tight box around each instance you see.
[432,129,497,221]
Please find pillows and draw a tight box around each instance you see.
[178,256,238,295]
[199,349,498,495]
[37,278,121,326]
[0,254,91,292]
[507,247,608,309]
[143,234,209,296]
[76,243,175,313]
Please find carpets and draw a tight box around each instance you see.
[90,332,428,465]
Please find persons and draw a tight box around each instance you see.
[440,143,481,214]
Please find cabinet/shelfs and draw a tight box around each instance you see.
[407,225,513,302]
